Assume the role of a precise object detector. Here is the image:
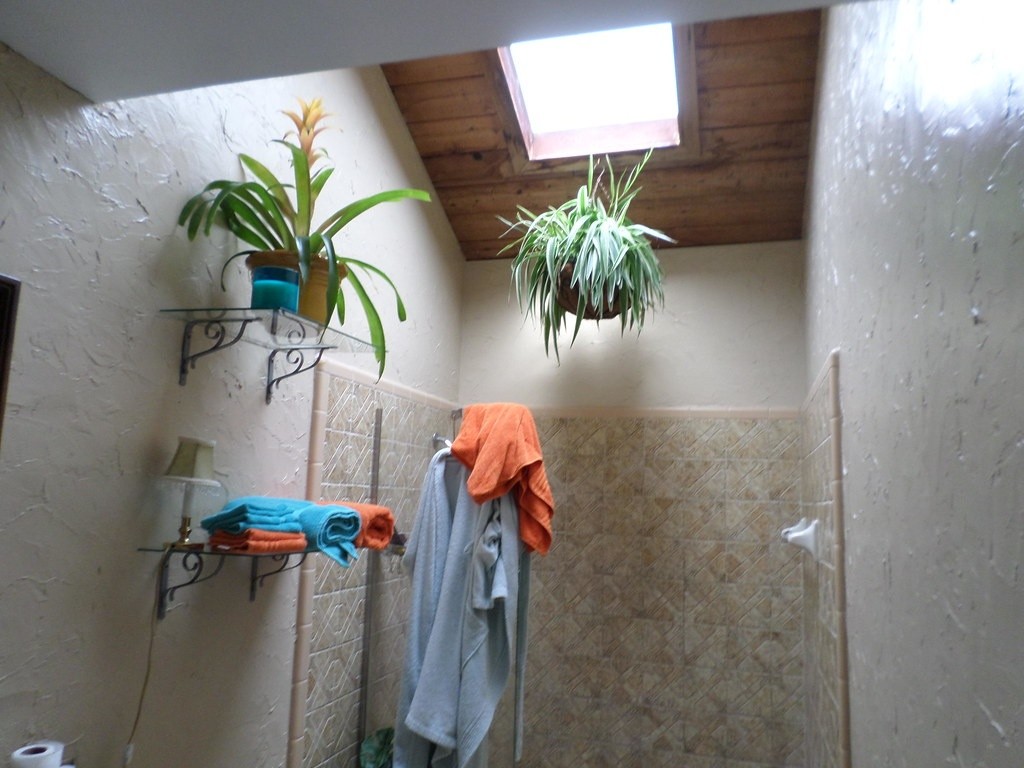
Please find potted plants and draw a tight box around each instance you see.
[495,150,679,369]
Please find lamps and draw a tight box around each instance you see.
[163,436,221,550]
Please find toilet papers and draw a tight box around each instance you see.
[9,738,66,768]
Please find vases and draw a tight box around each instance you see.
[248,248,349,320]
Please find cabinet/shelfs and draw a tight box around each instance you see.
[136,306,402,620]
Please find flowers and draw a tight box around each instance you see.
[178,99,431,382]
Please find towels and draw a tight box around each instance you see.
[450,401,556,559]
[199,501,304,535]
[311,500,396,550]
[390,447,521,768]
[222,496,363,569]
[208,527,308,555]
